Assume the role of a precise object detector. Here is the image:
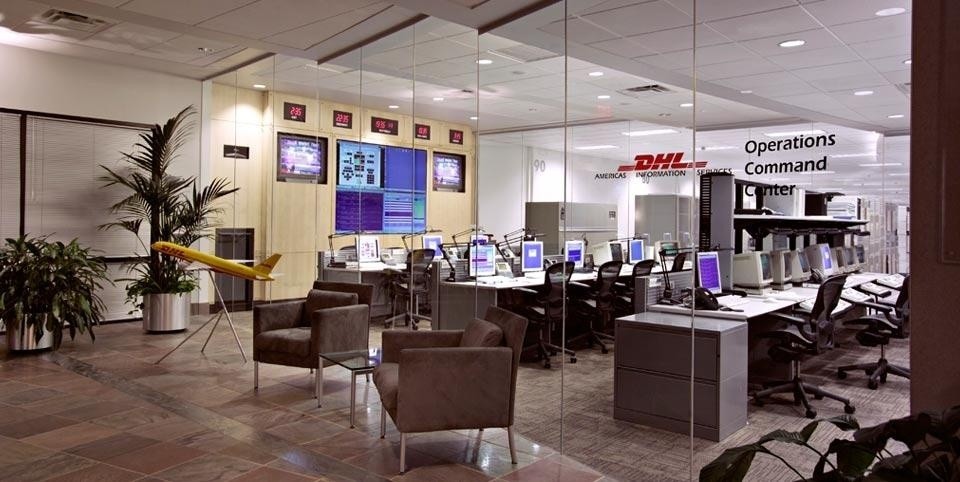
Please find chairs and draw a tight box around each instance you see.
[254,280,369,399]
[386,248,910,420]
[373,304,530,475]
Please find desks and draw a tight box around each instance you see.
[318,349,382,427]
[318,252,406,319]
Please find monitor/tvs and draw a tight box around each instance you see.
[422,236,446,259]
[336,139,427,234]
[278,134,327,185]
[521,241,545,272]
[731,245,865,293]
[592,241,625,271]
[564,240,584,268]
[468,243,497,278]
[692,250,724,296]
[655,240,681,262]
[433,154,464,193]
[628,240,645,264]
[354,236,383,264]
[469,235,490,246]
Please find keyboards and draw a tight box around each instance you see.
[712,295,749,309]
[476,275,517,285]
[524,271,547,279]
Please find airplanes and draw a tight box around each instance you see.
[150,241,284,283]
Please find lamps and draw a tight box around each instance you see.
[328,226,542,268]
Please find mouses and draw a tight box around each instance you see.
[764,298,779,304]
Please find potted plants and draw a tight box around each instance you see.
[96,104,241,332]
[0,232,115,351]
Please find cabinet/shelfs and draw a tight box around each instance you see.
[614,312,748,443]
